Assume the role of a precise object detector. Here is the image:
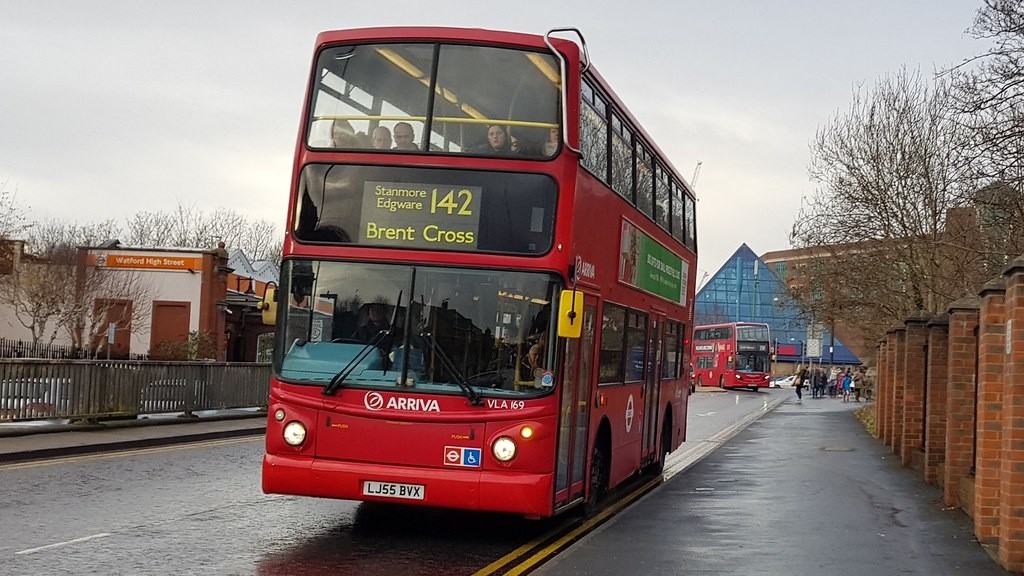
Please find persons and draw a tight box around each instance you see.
[809,365,872,402]
[330,120,355,147]
[503,325,518,338]
[392,122,420,150]
[372,126,392,150]
[520,343,545,381]
[351,304,399,351]
[479,125,508,152]
[546,128,558,155]
[794,364,806,403]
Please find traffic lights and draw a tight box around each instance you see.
[777,342,780,353]
[804,343,806,355]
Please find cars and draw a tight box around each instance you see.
[2,378,72,418]
[141,378,209,412]
[689,362,695,393]
[770,375,809,391]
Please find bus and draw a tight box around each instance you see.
[693,322,771,392]
[257,28,696,533]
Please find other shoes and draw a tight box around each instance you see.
[847,399,849,401]
[797,399,801,401]
[815,396,817,399]
[869,398,870,400]
[820,395,821,397]
[855,398,857,400]
[866,398,868,401]
[813,396,814,398]
[796,402,802,405]
[857,399,860,402]
[844,400,846,402]
